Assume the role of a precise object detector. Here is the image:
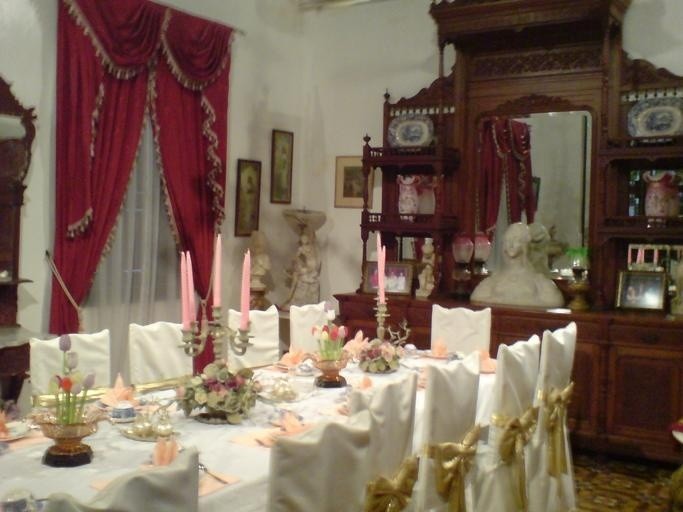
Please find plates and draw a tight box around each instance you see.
[626,98,682,144]
[388,117,434,153]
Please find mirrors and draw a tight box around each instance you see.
[473,93,596,283]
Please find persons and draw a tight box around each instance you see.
[528,219,550,275]
[287,232,321,308]
[470,222,566,308]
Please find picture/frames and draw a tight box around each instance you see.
[232,160,262,240]
[268,129,296,204]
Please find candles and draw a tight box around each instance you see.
[178,232,255,336]
